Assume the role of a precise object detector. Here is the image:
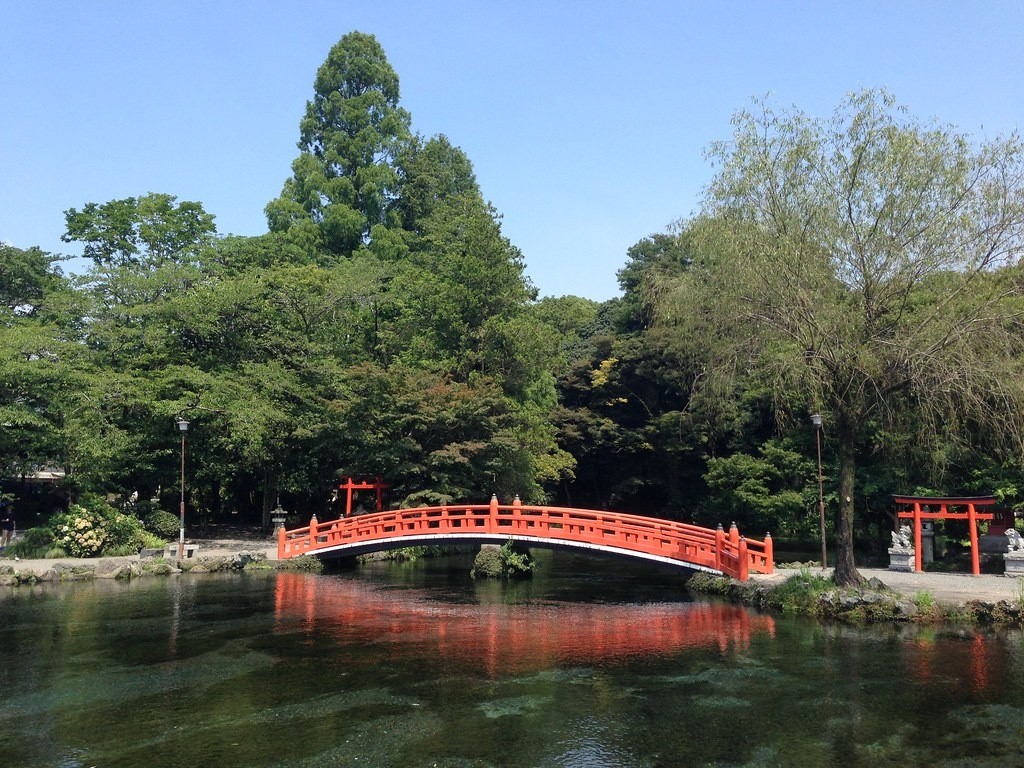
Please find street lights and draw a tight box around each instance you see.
[810,412,827,570]
[176,418,191,561]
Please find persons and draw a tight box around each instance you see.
[0,505,15,546]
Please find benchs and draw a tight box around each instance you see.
[169,544,199,558]
